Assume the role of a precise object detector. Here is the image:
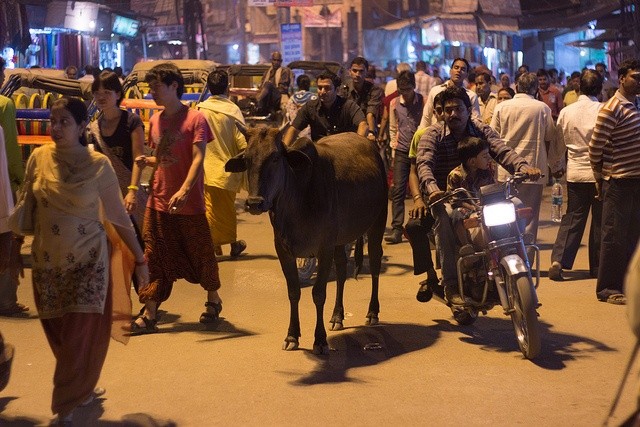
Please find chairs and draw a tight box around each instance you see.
[548,261,564,281]
[444,283,470,306]
[416,278,437,303]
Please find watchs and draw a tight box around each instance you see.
[419,171,545,359]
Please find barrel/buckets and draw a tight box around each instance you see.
[0,343,15,391]
[78,386,106,406]
[230,240,247,257]
[50,409,73,427]
[601,294,628,306]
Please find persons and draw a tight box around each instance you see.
[546,68,607,279]
[415,64,442,99]
[497,69,516,90]
[406,93,447,304]
[87,69,155,321]
[385,71,423,248]
[419,83,541,199]
[495,87,517,105]
[534,68,562,124]
[564,73,585,106]
[447,132,496,204]
[418,57,480,129]
[592,62,619,105]
[474,72,495,127]
[337,58,383,139]
[384,62,414,97]
[65,62,84,84]
[18,99,146,425]
[247,52,292,110]
[193,70,246,259]
[1,57,28,318]
[516,67,530,79]
[281,72,368,155]
[286,76,315,142]
[375,88,399,149]
[589,65,639,307]
[491,73,558,278]
[121,56,224,333]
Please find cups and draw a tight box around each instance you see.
[397,86,414,91]
[596,68,605,73]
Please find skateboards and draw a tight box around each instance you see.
[225,119,388,353]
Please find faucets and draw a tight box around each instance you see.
[120,314,160,334]
[200,298,222,324]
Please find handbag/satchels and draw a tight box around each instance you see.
[413,193,422,201]
[136,261,147,267]
[127,186,140,192]
